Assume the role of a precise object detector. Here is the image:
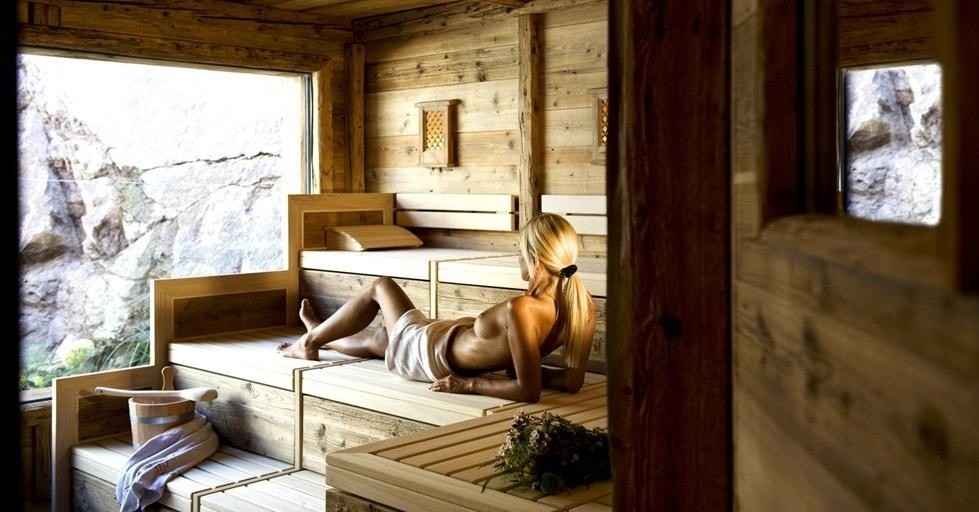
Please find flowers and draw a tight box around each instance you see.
[492,407,608,497]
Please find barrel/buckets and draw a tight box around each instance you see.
[128,395,194,452]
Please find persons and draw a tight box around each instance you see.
[276,212,597,403]
